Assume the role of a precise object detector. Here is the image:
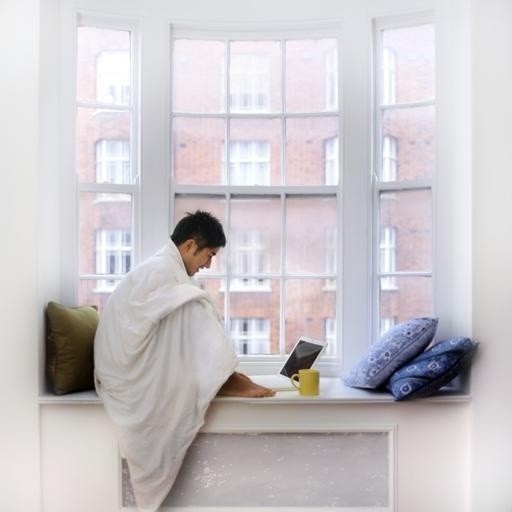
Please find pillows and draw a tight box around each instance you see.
[46,301,99,397]
[343,315,481,402]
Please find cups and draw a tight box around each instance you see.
[290,368,321,397]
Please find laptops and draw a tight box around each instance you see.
[246,336,328,392]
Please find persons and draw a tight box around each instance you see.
[92,210,277,400]
